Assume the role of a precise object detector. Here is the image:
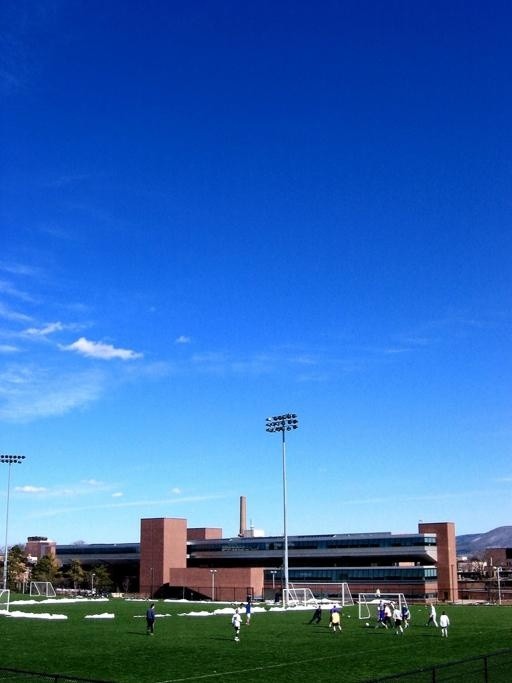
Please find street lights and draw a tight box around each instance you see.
[92,574,95,593]
[210,570,216,601]
[266,415,298,604]
[0,456,25,591]
[493,566,503,605]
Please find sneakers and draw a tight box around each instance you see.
[147,630,154,636]
[234,635,241,642]
[306,620,449,639]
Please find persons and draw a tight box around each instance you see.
[331,609,342,633]
[328,605,344,627]
[309,605,322,624]
[231,610,243,642]
[425,601,439,627]
[374,599,410,635]
[145,602,157,635]
[439,611,450,638]
[247,593,252,603]
[244,601,252,626]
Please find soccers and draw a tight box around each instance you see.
[366,623,369,627]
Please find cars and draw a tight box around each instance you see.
[80,589,109,598]
